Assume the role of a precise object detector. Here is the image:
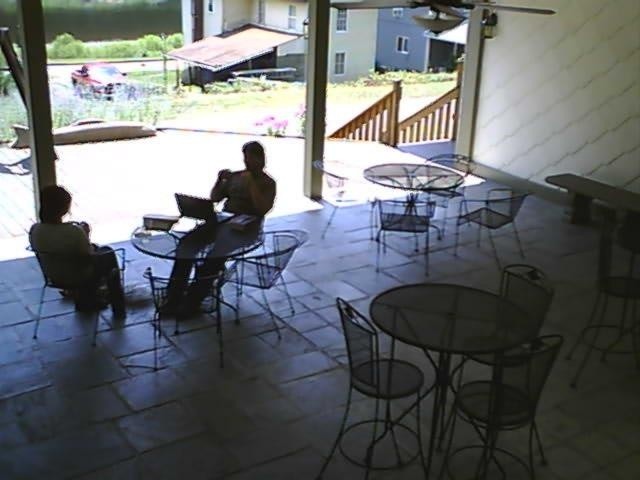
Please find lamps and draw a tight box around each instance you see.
[410,6,465,37]
[479,13,500,39]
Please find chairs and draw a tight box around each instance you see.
[564,218,639,390]
[314,296,430,478]
[24,235,129,352]
[438,332,566,477]
[141,266,242,372]
[219,227,310,340]
[310,150,535,272]
[436,264,556,467]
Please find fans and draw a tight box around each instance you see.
[326,0,558,19]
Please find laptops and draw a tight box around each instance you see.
[173,192,240,227]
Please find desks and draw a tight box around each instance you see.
[125,215,245,339]
[362,279,539,479]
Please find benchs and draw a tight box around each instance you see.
[544,173,639,226]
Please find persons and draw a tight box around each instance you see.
[156,141,275,317]
[29,186,127,319]
